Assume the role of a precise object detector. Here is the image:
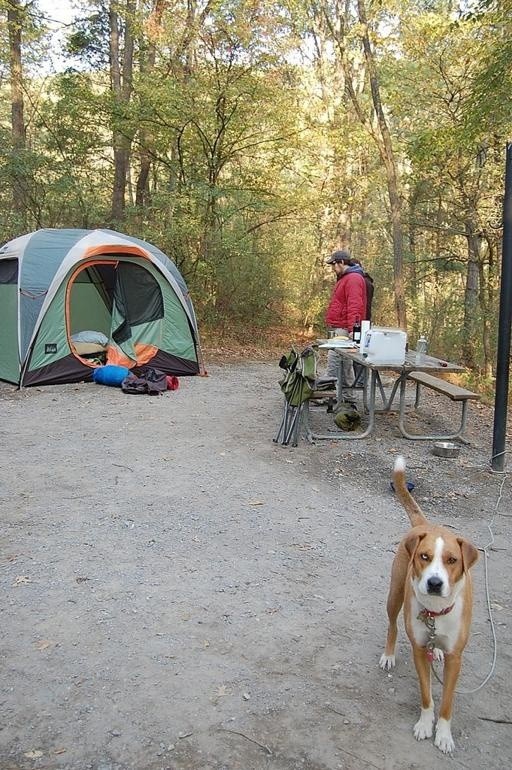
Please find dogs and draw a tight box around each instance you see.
[377,454,479,756]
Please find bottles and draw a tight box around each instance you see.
[416,334,426,367]
[352,314,362,344]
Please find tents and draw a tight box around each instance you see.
[0,225,202,388]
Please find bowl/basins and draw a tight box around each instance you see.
[433,441,462,459]
[389,479,415,492]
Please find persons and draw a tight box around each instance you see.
[310,251,367,405]
[350,258,374,390]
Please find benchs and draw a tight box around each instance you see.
[270,331,483,440]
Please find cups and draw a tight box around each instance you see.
[327,330,338,339]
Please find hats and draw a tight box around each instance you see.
[326,251,349,264]
